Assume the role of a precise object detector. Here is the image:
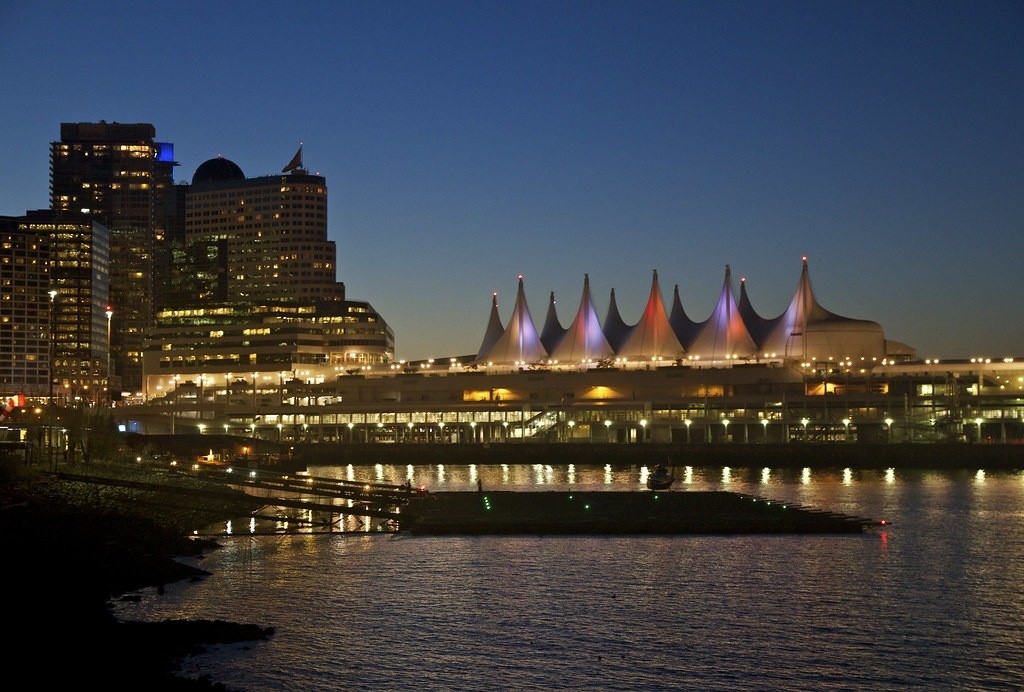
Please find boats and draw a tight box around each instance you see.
[646,464,676,490]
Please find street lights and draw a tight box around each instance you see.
[842,418,852,441]
[760,419,770,443]
[722,418,730,442]
[249,423,257,438]
[603,420,614,443]
[276,423,284,442]
[502,422,509,439]
[347,422,354,444]
[974,417,986,442]
[684,419,692,442]
[223,424,228,435]
[469,421,477,443]
[567,420,576,442]
[437,421,445,443]
[104,310,113,404]
[48,288,58,408]
[408,422,416,444]
[639,419,649,444]
[801,417,809,439]
[198,424,205,434]
[884,418,894,443]
